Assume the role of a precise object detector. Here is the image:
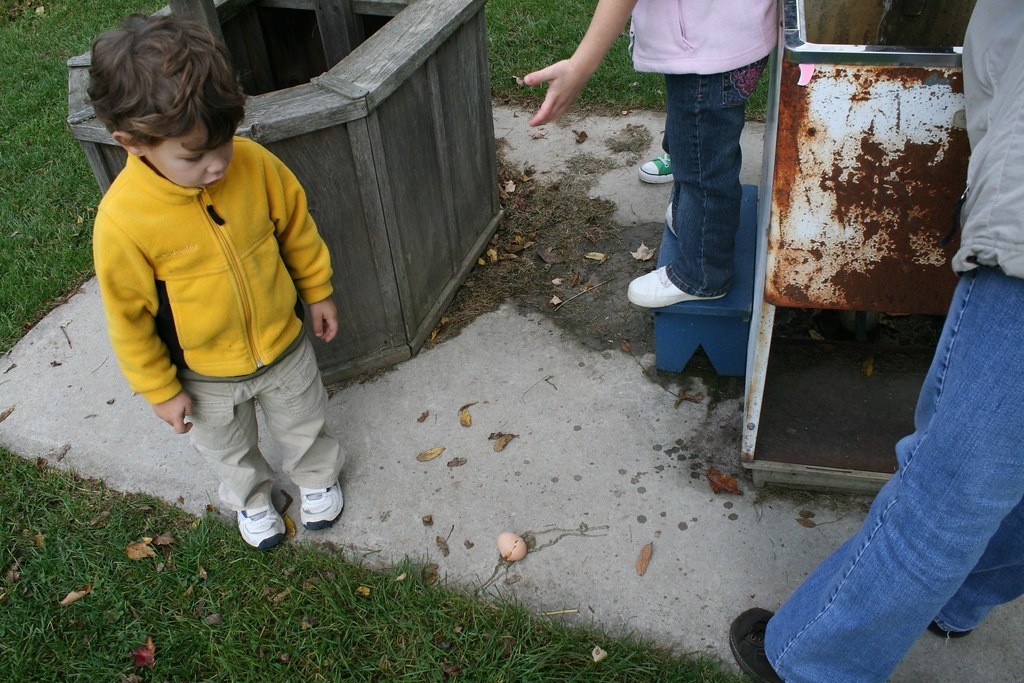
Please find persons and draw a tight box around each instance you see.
[725,0,1024,682]
[85,15,345,550]
[524,0,779,307]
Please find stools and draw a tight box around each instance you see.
[650,185,759,377]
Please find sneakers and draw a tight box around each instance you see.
[298,478,344,531]
[638,153,674,183]
[729,608,786,683]
[666,202,677,237]
[927,620,973,638]
[237,497,286,548]
[628,266,727,308]
